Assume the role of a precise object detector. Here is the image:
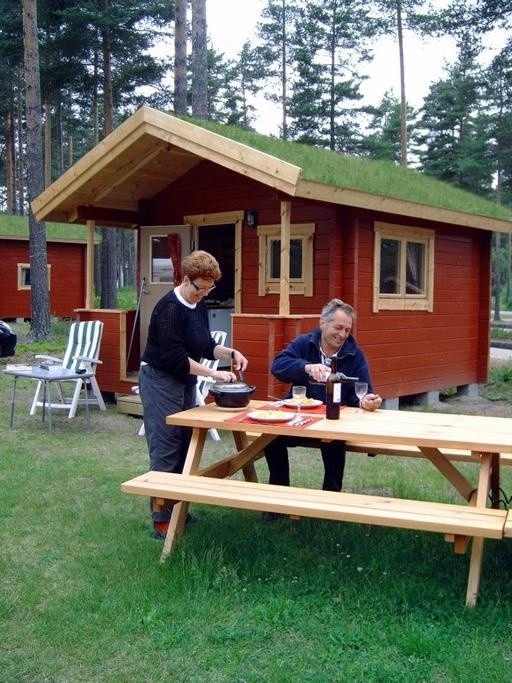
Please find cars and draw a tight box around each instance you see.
[0,317,18,360]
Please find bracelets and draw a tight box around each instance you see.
[232,350,236,360]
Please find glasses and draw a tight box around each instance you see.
[189,279,217,292]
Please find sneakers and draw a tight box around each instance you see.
[260,511,288,521]
[152,509,200,539]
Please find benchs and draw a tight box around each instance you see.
[118,433,512,609]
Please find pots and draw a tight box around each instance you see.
[209,385,256,407]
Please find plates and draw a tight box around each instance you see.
[247,410,297,423]
[282,398,324,408]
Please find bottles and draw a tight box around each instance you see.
[310,358,360,419]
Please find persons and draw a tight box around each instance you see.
[259,296,383,527]
[139,247,250,540]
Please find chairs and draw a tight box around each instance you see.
[28,319,107,418]
[129,329,227,441]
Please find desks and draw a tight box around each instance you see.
[157,399,511,605]
[1,365,95,436]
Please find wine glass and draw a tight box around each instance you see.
[291,386,308,425]
[354,381,368,414]
